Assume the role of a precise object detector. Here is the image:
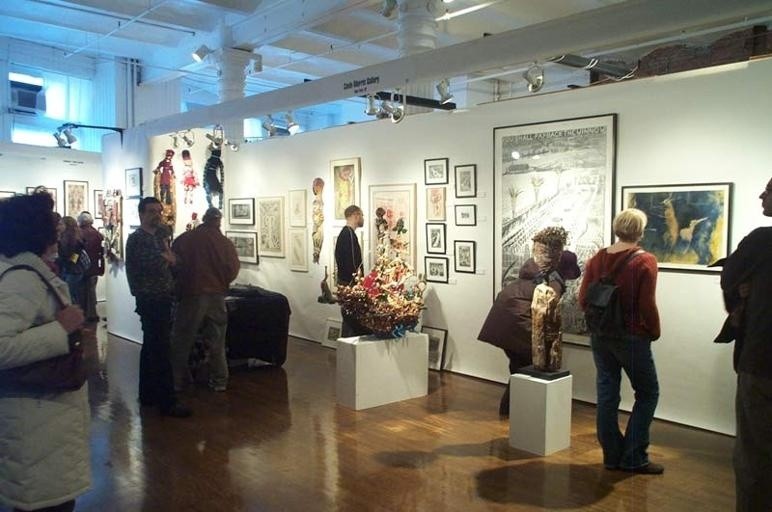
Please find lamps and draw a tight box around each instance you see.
[522,63,545,93]
[364,85,406,124]
[191,44,214,61]
[169,126,223,149]
[54,123,123,147]
[261,113,302,137]
[436,77,453,105]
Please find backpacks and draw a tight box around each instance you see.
[584,247,646,341]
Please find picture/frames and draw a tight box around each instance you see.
[1,180,111,254]
[621,183,732,274]
[423,158,477,283]
[124,167,143,228]
[321,318,343,350]
[223,189,308,273]
[368,183,417,277]
[493,113,617,348]
[421,326,448,371]
[331,158,362,231]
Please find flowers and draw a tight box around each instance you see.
[334,206,426,311]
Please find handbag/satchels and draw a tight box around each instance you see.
[0,337,87,394]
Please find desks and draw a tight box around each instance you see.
[335,330,428,411]
[225,282,291,371]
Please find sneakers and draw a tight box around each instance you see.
[208,381,227,393]
[176,383,195,393]
[162,407,191,418]
[623,462,664,474]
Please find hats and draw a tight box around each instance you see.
[558,251,581,280]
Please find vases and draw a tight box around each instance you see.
[356,311,419,339]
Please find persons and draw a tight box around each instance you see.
[181,150,196,206]
[203,147,225,207]
[579,208,665,475]
[154,150,177,203]
[311,178,325,262]
[722,177,772,510]
[126,198,194,417]
[478,250,581,415]
[0,192,104,512]
[336,205,364,334]
[171,207,241,393]
